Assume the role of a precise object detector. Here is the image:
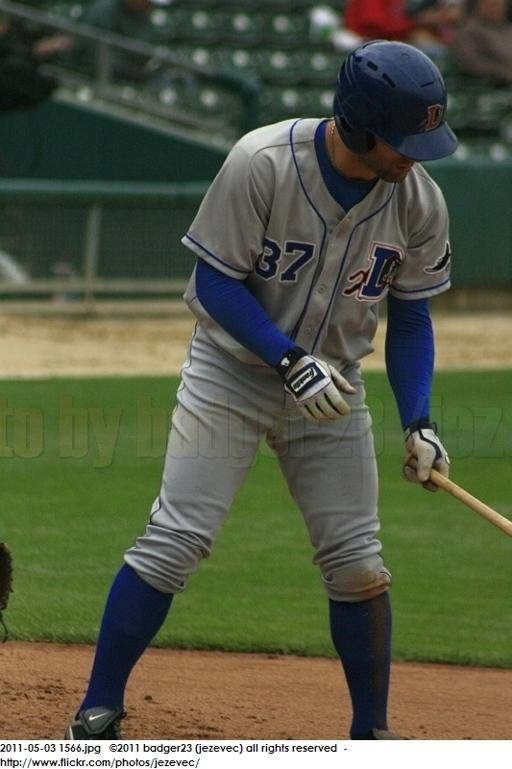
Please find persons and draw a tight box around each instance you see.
[343,0,512,88]
[64,39,461,740]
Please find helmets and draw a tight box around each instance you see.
[334,40,459,162]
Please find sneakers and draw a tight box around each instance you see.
[64,705,126,739]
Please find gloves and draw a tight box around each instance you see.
[401,420,450,491]
[283,351,356,422]
